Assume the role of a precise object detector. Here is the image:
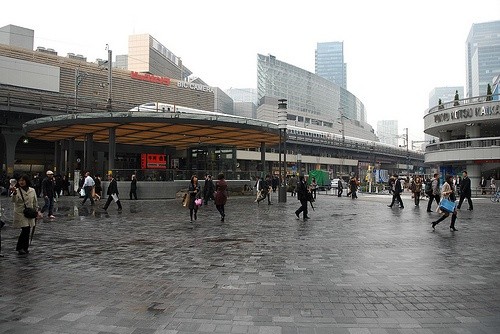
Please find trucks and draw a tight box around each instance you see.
[309,169,332,191]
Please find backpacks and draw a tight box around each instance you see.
[424,180,435,194]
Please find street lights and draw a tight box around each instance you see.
[277,98,287,203]
[399,145,409,191]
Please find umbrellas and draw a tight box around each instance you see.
[487,84,493,100]
[454,91,460,106]
[439,99,441,105]
[30,208,41,244]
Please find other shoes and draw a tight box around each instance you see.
[38,211,44,217]
[47,215,56,219]
[387,205,392,208]
[399,206,404,208]
[18,249,29,254]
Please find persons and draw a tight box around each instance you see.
[337,176,358,199]
[253,175,279,205]
[101,170,122,211]
[431,176,458,231]
[188,175,201,221]
[294,174,310,220]
[129,175,138,200]
[452,171,496,211]
[33,172,42,198]
[203,174,215,204]
[39,170,56,219]
[53,176,69,202]
[387,173,440,212]
[11,174,39,255]
[215,173,227,222]
[78,172,102,206]
[310,177,317,199]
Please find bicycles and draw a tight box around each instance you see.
[490,187,500,203]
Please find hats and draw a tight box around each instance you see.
[46,171,54,175]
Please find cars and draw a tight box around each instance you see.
[331,179,348,189]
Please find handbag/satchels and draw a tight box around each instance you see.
[438,198,456,213]
[195,198,202,206]
[111,193,118,203]
[81,188,86,196]
[182,192,190,208]
[23,207,38,219]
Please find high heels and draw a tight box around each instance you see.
[430,222,436,230]
[449,225,458,231]
